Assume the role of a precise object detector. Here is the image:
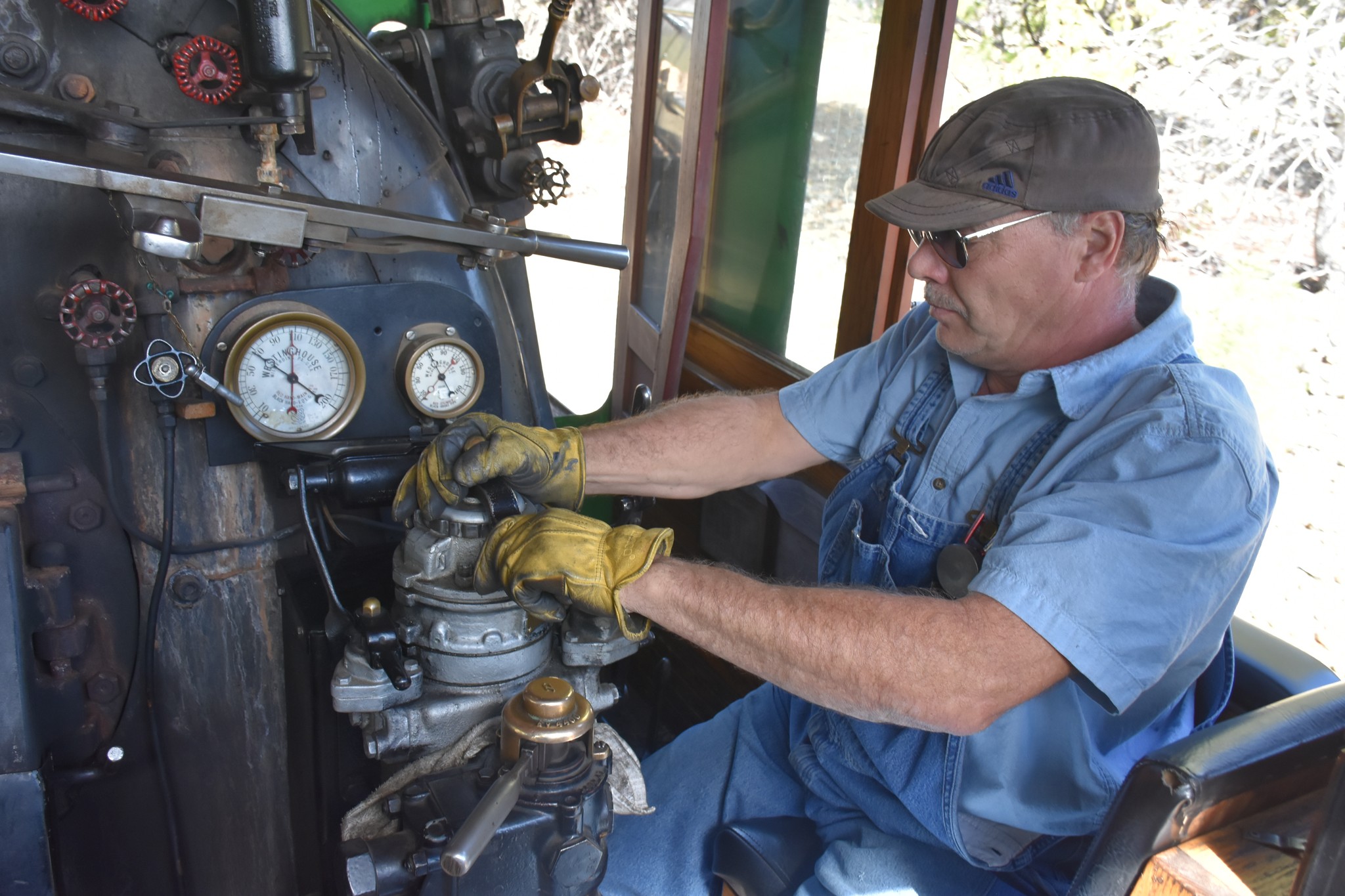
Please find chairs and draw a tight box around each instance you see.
[710,613,1344,896]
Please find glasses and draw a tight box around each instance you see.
[907,211,1058,269]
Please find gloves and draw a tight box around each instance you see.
[393,412,586,512]
[474,509,673,638]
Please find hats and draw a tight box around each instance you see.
[865,78,1165,229]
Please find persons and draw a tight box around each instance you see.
[391,76,1279,896]
